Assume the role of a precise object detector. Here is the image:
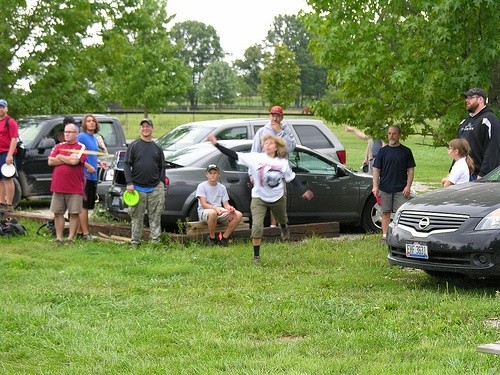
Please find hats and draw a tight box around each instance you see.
[461,88,486,99]
[0,100,7,106]
[270,106,282,115]
[140,118,154,128]
[206,164,220,173]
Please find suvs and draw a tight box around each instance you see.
[12,113,127,212]
[96,117,347,200]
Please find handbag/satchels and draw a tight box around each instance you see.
[0,218,26,236]
[15,141,23,171]
[48,217,70,236]
[363,164,368,172]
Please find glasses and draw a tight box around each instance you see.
[64,131,76,134]
[466,96,479,99]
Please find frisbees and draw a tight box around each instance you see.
[123,189,139,206]
[1,163,16,177]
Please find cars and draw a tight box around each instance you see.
[385,161,500,283]
[105,139,418,236]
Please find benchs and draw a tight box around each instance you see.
[5,210,55,235]
[187,217,250,244]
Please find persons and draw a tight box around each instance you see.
[346,126,387,174]
[57,115,82,143]
[458,88,500,181]
[77,115,109,242]
[196,164,242,248]
[123,119,166,248]
[441,138,475,186]
[251,106,297,227]
[48,123,88,246]
[372,125,416,243]
[0,99,19,212]
[208,134,313,261]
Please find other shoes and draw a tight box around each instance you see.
[64,239,73,246]
[55,240,62,247]
[207,235,217,247]
[254,257,260,264]
[83,234,92,241]
[218,233,228,247]
[129,241,141,251]
[77,233,83,239]
[279,223,289,241]
[151,237,159,244]
